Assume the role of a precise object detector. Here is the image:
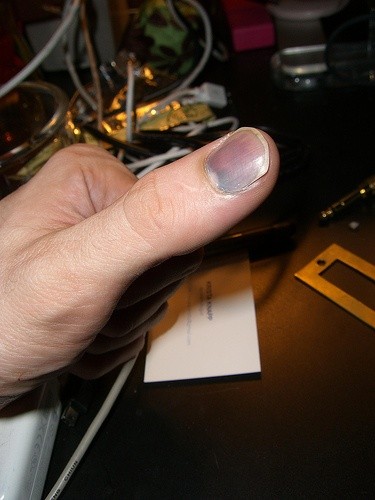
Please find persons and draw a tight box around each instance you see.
[0,125,281,409]
